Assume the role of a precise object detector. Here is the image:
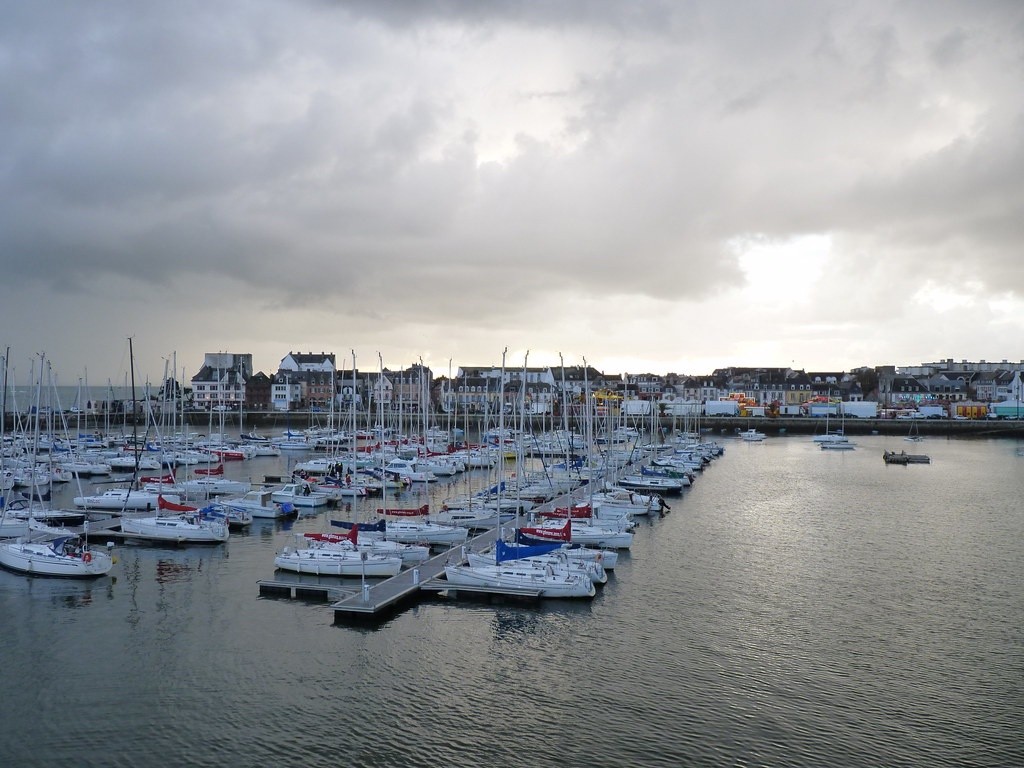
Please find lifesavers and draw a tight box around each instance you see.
[596,553,604,563]
[557,489,564,496]
[56,468,61,473]
[82,551,91,562]
[453,462,456,466]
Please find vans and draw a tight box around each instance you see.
[985,412,998,420]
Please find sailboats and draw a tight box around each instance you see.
[903,411,925,442]
[812,385,849,443]
[0,335,726,601]
[820,403,858,449]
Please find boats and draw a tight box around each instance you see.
[738,429,768,442]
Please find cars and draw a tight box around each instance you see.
[927,414,946,420]
[1005,415,1021,419]
[915,413,927,419]
[953,414,968,420]
[897,414,913,419]
[804,412,859,420]
